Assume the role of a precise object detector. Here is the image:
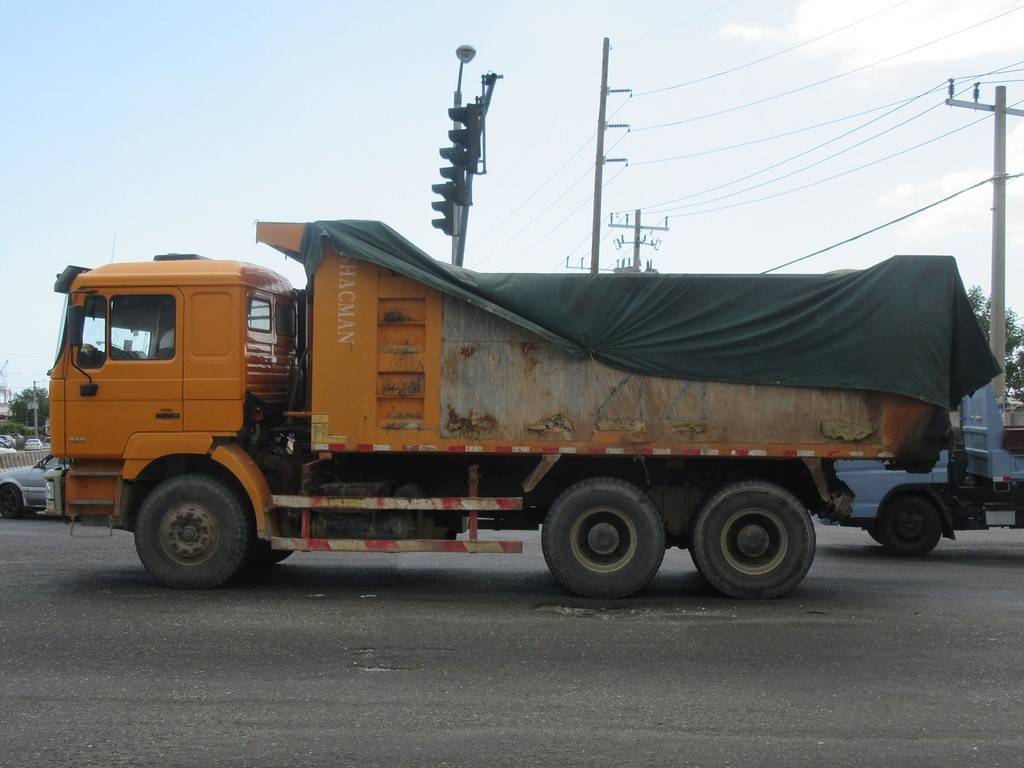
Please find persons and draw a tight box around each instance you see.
[111,297,176,361]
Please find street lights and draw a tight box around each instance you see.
[452,44,500,269]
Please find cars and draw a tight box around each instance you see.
[0,434,15,447]
[43,437,51,449]
[25,439,45,450]
[0,451,69,519]
[0,437,18,453]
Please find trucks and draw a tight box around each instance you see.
[810,374,1023,558]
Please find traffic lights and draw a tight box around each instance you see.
[438,143,475,208]
[432,181,454,239]
[447,99,483,176]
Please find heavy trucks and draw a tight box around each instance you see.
[43,214,951,603]
